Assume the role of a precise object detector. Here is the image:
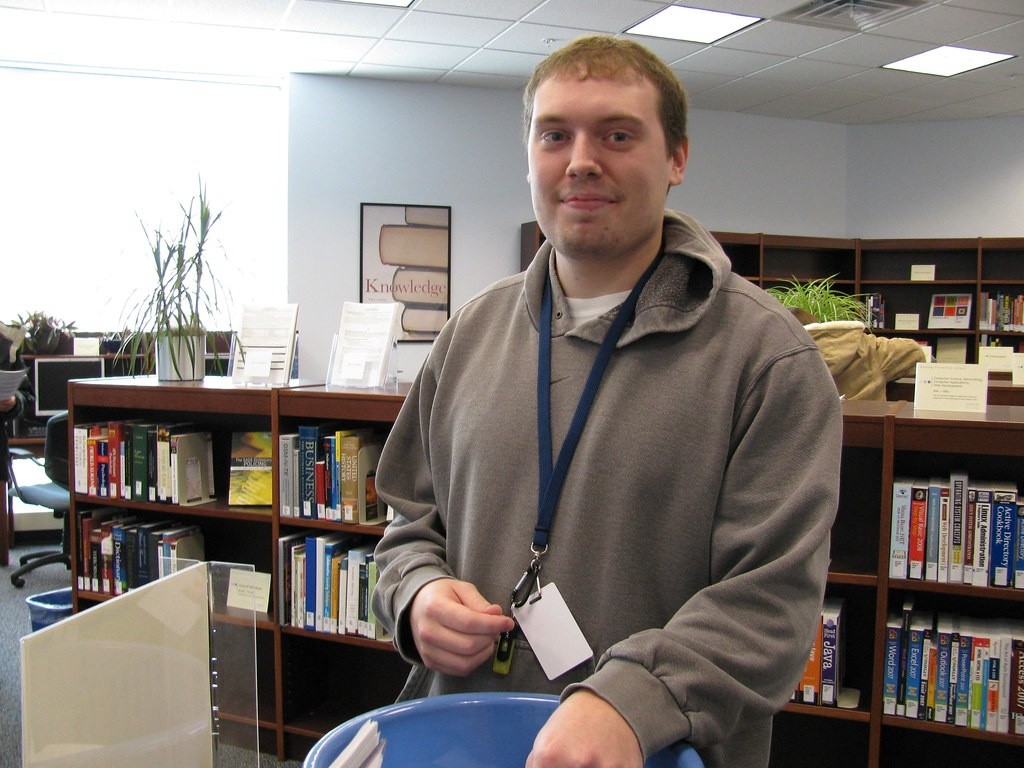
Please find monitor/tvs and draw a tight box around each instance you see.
[35,357,105,415]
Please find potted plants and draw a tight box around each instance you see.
[112,172,247,382]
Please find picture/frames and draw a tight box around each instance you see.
[359,202,452,342]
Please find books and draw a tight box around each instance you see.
[278,426,394,642]
[866,292,1024,363]
[74,422,272,620]
[882,602,1024,737]
[790,595,845,708]
[889,474,1024,590]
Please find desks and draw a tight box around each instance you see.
[0,331,238,569]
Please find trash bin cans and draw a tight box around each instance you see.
[24,587,73,632]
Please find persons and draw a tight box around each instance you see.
[785,306,927,403]
[368,37,844,768]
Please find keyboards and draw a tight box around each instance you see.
[28,425,47,438]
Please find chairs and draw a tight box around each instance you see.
[9,410,70,590]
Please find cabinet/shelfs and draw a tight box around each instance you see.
[69,222,1024,768]
